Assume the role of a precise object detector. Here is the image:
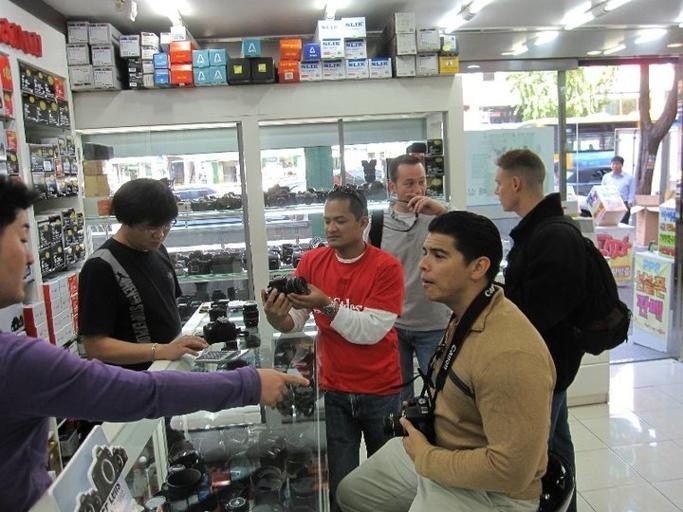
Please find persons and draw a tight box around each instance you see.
[492,148,590,512]
[260,183,407,511]
[601,155,637,225]
[334,209,559,512]
[0,172,311,512]
[362,154,454,398]
[76,177,211,372]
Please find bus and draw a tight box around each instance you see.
[555,116,642,195]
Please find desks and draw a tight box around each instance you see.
[630,251,683,356]
[592,223,635,288]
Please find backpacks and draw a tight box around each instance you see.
[535,217,631,354]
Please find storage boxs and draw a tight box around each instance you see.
[586,184,627,225]
[629,194,660,248]
[658,198,676,258]
[556,185,578,216]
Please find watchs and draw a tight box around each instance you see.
[321,300,337,317]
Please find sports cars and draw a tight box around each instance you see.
[170,186,216,198]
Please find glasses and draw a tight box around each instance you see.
[328,183,366,208]
[138,218,176,232]
[380,199,417,231]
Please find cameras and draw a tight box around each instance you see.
[111,447,128,473]
[79,490,104,512]
[264,275,309,303]
[160,177,242,212]
[357,180,387,201]
[203,320,237,345]
[175,295,203,313]
[273,375,315,417]
[383,396,436,444]
[263,184,328,207]
[169,250,248,276]
[168,450,205,470]
[92,446,122,503]
[194,282,235,302]
[243,303,259,328]
[209,302,227,321]
[268,235,326,270]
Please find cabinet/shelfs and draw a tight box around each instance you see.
[28,300,332,512]
[256,108,450,299]
[0,2,90,482]
[75,129,256,308]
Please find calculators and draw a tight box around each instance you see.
[194,348,250,364]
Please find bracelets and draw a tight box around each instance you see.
[151,342,158,362]
